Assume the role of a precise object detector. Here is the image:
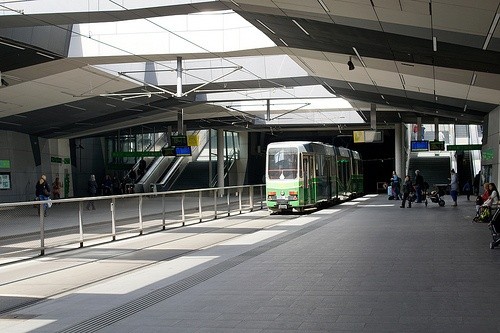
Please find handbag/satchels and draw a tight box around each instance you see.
[476,196,484,205]
[33,198,39,208]
[481,207,490,223]
[48,195,53,208]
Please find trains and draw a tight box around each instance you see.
[265,141,381,214]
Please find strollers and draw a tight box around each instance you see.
[429,182,448,206]
[488,208,500,249]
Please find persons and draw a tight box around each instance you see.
[391,171,398,183]
[463,181,471,200]
[476,183,499,211]
[86,175,97,210]
[35,175,51,216]
[449,170,458,205]
[400,175,413,208]
[106,158,147,196]
[414,170,423,203]
[52,178,61,205]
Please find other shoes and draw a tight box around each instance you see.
[92,206,96,210]
[400,206,405,208]
[407,207,410,208]
[86,204,91,210]
[451,201,457,206]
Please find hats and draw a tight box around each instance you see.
[451,169,455,173]
